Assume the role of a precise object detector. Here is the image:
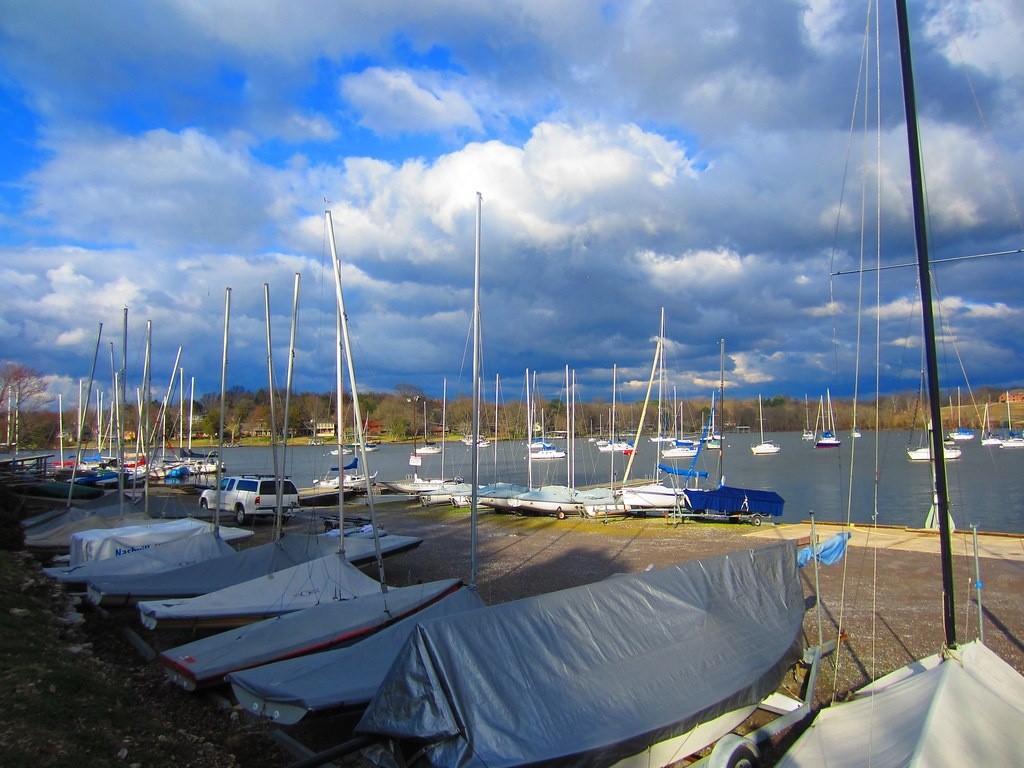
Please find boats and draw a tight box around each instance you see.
[0,0,1024,768]
